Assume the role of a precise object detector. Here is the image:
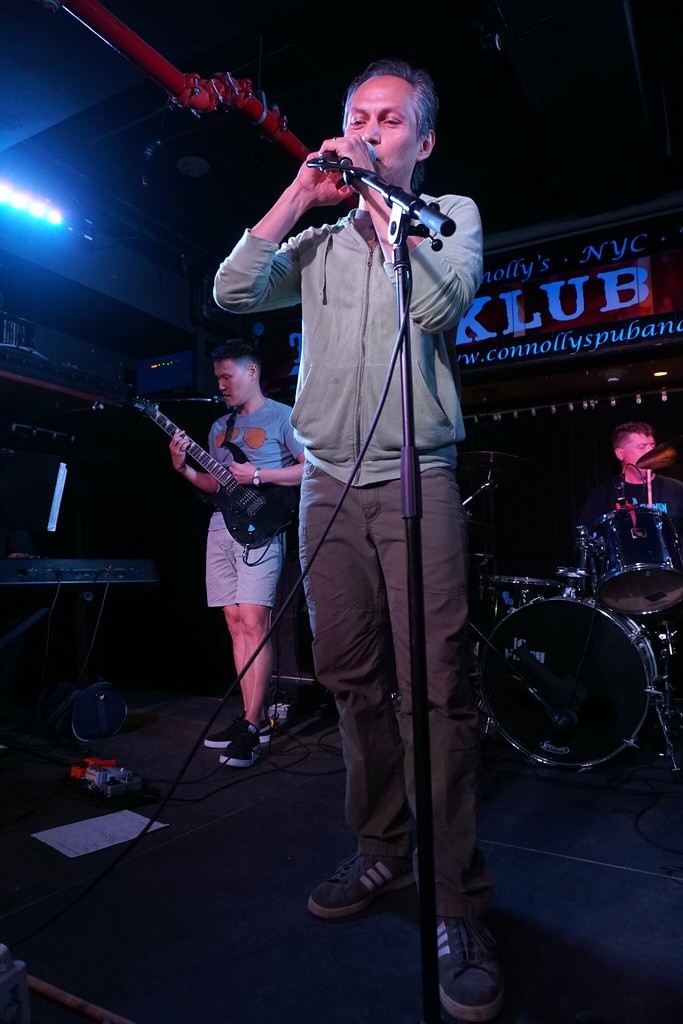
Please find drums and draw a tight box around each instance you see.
[475,576,565,619]
[476,595,659,771]
[579,504,683,617]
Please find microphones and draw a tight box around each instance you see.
[616,467,626,501]
[306,142,376,167]
[554,708,578,727]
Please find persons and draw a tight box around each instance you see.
[211,62,511,1021]
[168,341,305,769]
[572,420,683,562]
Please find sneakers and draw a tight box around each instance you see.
[435,915,505,1020]
[203,710,270,748]
[219,720,260,767]
[308,851,409,918]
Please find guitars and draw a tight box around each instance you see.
[130,396,294,550]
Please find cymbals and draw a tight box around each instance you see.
[456,449,538,484]
[636,435,683,473]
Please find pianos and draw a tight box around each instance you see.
[0,553,164,603]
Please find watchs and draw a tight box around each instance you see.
[252,467,262,487]
